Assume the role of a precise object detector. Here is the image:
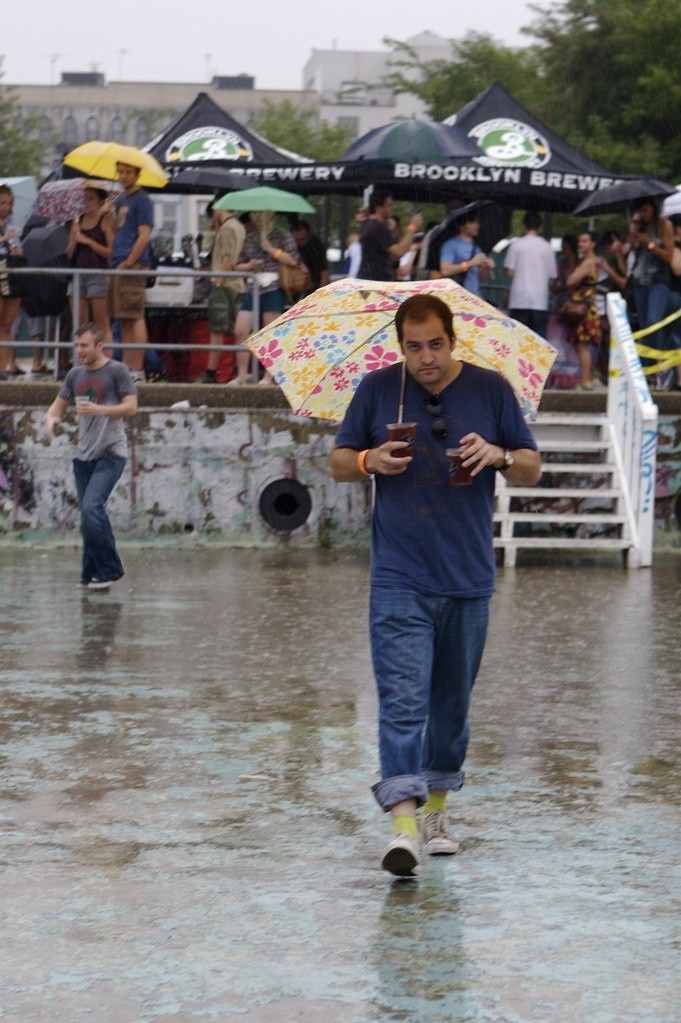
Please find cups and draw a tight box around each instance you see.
[75,395,90,417]
[387,422,418,460]
[446,447,474,487]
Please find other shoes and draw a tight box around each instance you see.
[259,376,275,386]
[76,577,91,588]
[88,577,114,589]
[0,371,8,380]
[229,373,248,385]
[193,370,216,383]
[573,378,593,392]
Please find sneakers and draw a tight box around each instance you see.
[423,802,459,854]
[382,833,420,876]
[130,368,146,384]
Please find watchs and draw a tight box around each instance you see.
[496,449,513,471]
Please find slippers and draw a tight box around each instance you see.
[8,368,26,374]
[32,365,53,374]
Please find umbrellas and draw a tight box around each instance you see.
[0,176,38,233]
[33,178,126,220]
[63,141,170,204]
[240,278,560,422]
[168,168,258,193]
[342,111,486,213]
[428,200,492,269]
[572,182,676,235]
[212,186,315,239]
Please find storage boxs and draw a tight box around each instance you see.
[146,266,196,306]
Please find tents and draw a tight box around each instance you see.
[139,81,641,274]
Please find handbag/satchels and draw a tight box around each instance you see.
[556,299,587,323]
[277,231,312,293]
[442,270,466,286]
[144,251,159,287]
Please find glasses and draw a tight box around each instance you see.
[425,394,448,440]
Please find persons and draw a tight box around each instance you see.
[332,294,540,877]
[44,323,138,589]
[0,161,681,382]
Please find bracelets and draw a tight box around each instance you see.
[358,449,379,476]
[648,242,655,249]
[462,262,468,272]
[407,224,418,232]
[272,249,281,260]
